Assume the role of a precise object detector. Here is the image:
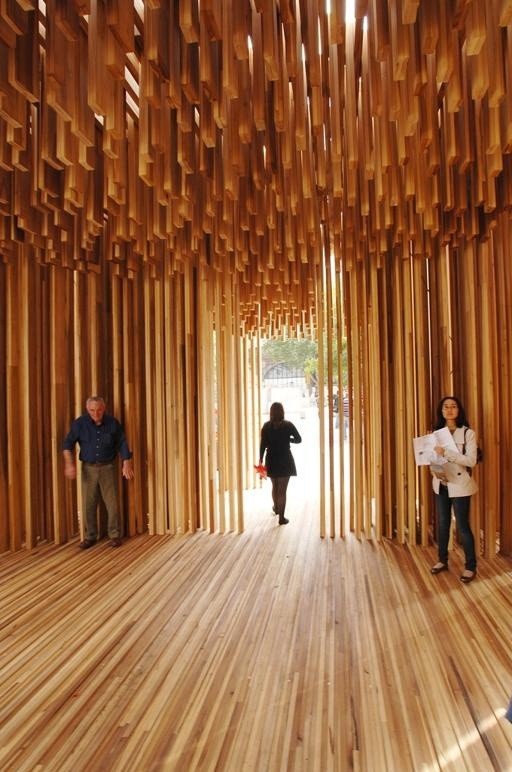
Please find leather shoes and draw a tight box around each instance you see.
[460,569,477,583]
[79,539,97,549]
[110,537,122,547]
[431,561,448,574]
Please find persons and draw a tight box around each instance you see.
[60,395,136,550]
[426,395,480,585]
[255,401,303,527]
[315,390,349,440]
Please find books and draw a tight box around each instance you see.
[411,425,462,467]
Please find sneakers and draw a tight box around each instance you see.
[279,518,290,525]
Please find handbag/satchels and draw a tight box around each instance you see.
[462,428,482,463]
